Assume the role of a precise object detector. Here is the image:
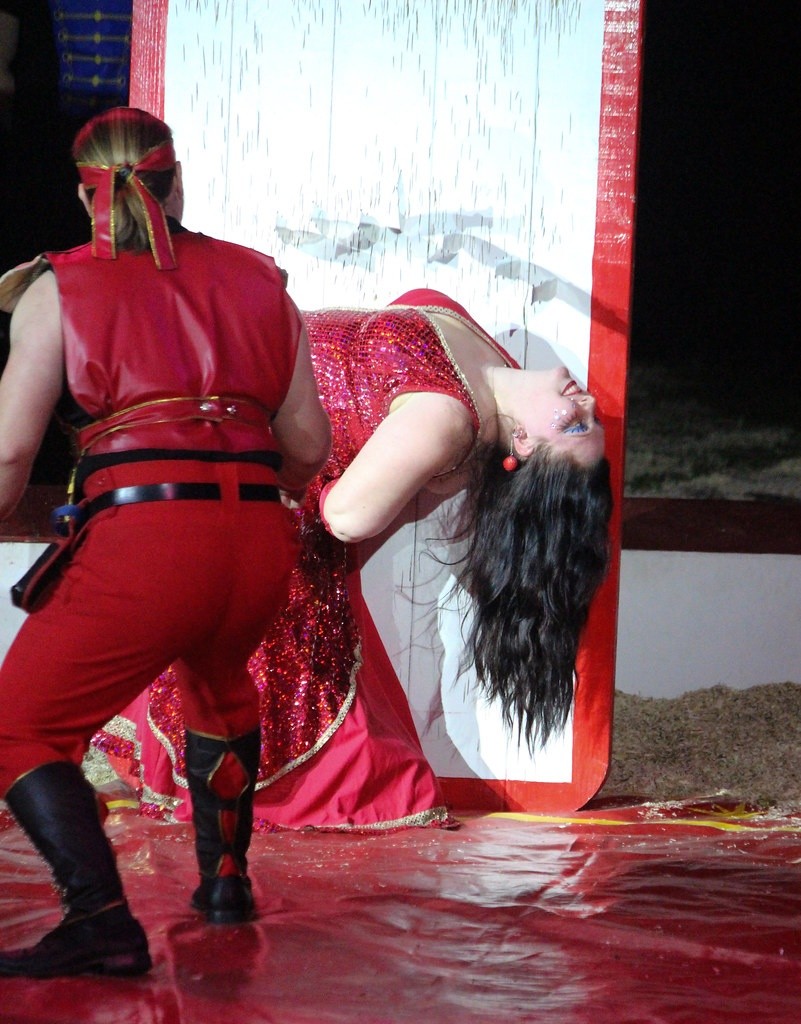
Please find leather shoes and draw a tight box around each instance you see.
[0,904,153,980]
[191,875,254,925]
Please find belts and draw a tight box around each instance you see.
[71,483,285,536]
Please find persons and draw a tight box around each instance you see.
[83,288,614,836]
[1,107,329,975]
